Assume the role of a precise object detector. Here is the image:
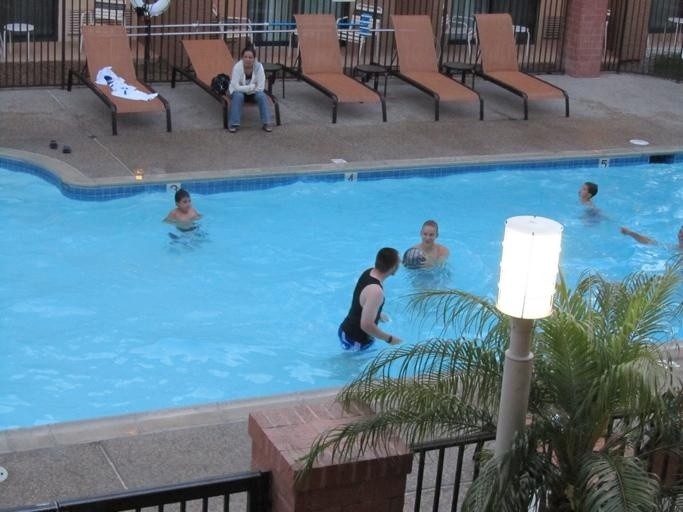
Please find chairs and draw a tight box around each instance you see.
[330,4,382,62]
[78,1,128,53]
[170,32,280,126]
[470,9,569,125]
[440,4,473,59]
[285,10,388,126]
[385,12,484,123]
[63,19,176,141]
[212,7,255,51]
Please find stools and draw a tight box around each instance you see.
[661,15,683,47]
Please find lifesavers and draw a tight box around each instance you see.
[128,0,169,20]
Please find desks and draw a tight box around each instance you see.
[508,24,532,59]
[261,61,281,89]
[443,60,481,93]
[353,63,389,91]
[1,20,36,62]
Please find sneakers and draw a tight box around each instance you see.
[228,125,240,133]
[262,124,273,132]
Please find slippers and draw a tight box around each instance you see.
[48,139,58,150]
[61,144,73,154]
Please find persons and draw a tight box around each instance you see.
[402,218,449,271]
[335,247,403,352]
[225,46,274,134]
[617,223,682,255]
[576,180,607,223]
[161,189,202,233]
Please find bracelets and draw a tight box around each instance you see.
[384,335,393,344]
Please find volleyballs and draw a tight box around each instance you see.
[403,248,426,269]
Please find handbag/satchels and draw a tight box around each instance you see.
[210,73,230,97]
